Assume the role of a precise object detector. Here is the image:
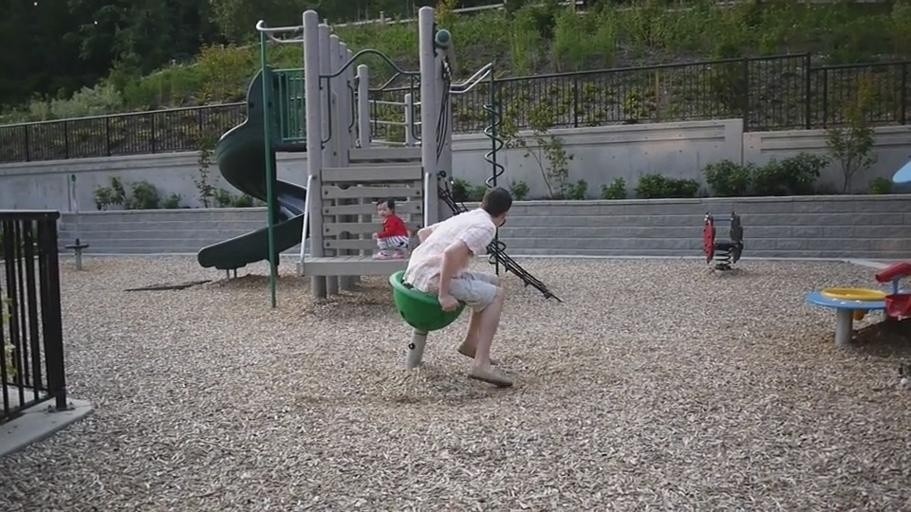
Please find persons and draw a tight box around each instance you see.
[368,198,414,258]
[398,186,514,386]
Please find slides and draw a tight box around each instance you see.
[196,65,309,268]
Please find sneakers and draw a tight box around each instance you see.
[467,366,513,386]
[455,339,498,365]
[372,250,391,260]
[391,251,404,259]
